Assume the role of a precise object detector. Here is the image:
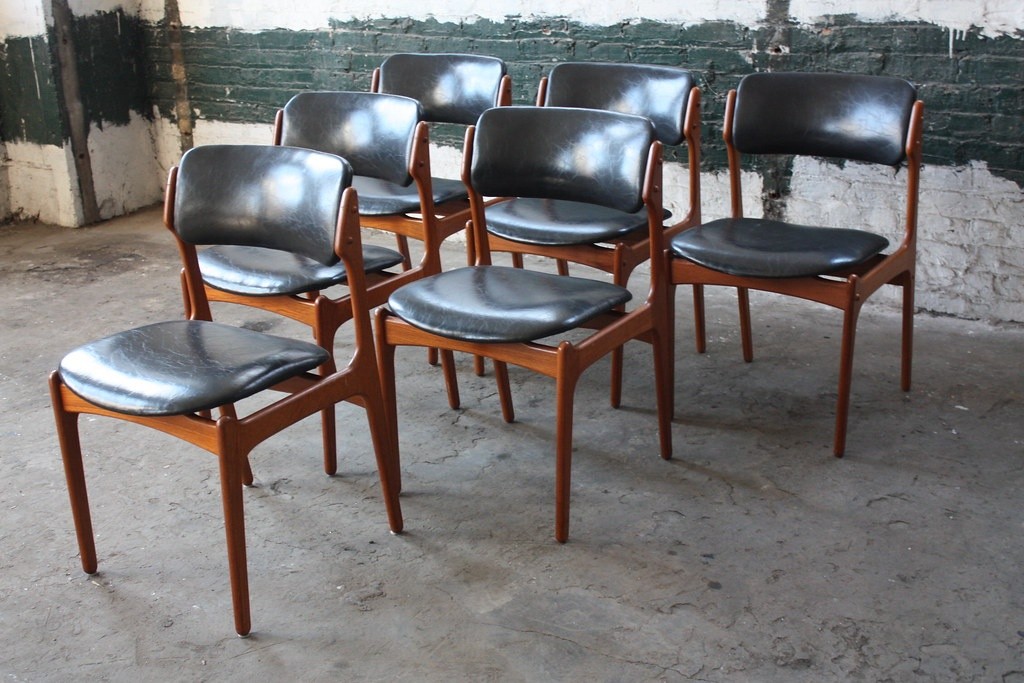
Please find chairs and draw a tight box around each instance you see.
[373,105,673,543]
[466,62,706,408]
[180,91,461,474]
[370,51,524,269]
[664,71,924,457]
[49,145,404,635]
